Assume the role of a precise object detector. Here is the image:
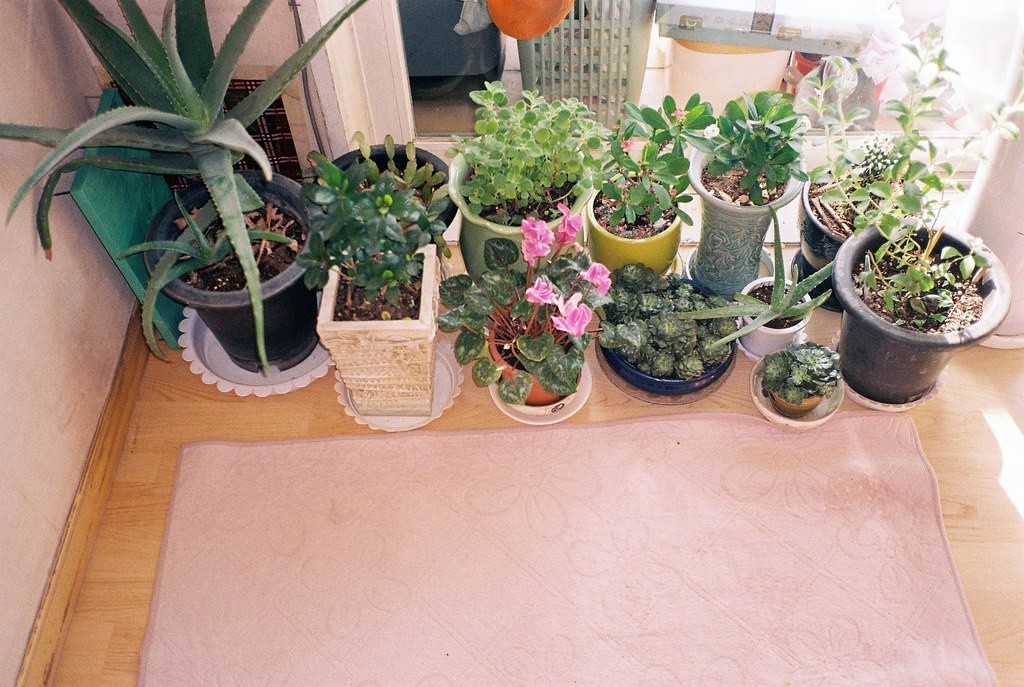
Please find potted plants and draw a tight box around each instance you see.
[293,174,466,432]
[793,135,923,313]
[686,91,806,291]
[444,80,613,285]
[811,32,1024,411]
[601,259,741,404]
[670,204,835,363]
[0,0,359,398]
[306,131,458,243]
[748,340,845,428]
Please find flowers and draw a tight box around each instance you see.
[593,94,716,223]
[442,212,613,402]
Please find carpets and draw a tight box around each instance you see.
[136,410,999,686]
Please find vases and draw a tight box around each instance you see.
[486,309,592,424]
[587,190,681,275]
